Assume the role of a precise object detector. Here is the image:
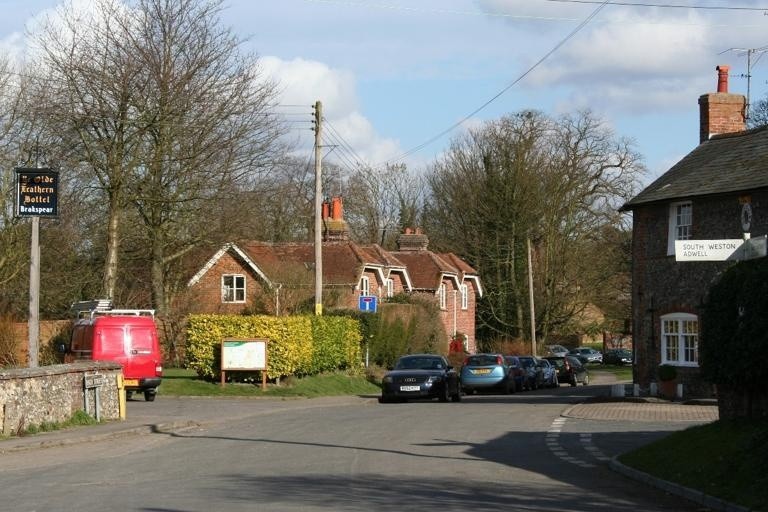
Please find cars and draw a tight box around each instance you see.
[379,342,633,403]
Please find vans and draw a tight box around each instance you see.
[56,304,165,403]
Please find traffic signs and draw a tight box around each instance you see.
[673,237,745,264]
[10,164,63,220]
